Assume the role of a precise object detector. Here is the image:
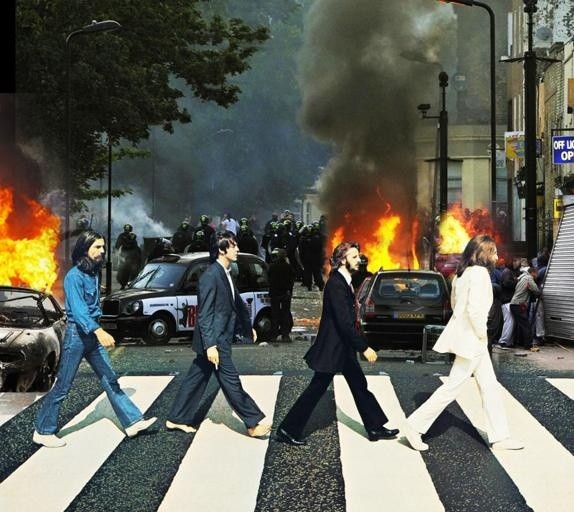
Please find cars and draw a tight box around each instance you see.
[0,285,68,392]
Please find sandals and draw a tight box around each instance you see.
[164,419,198,433]
[247,424,269,438]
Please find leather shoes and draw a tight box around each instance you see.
[32,429,66,447]
[275,425,307,447]
[368,427,400,442]
[124,416,158,438]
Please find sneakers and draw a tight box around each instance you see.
[492,438,525,450]
[404,424,429,452]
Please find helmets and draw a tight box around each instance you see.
[75,217,89,227]
[123,224,133,232]
[296,220,303,229]
[200,214,210,224]
[283,219,291,227]
[181,218,191,225]
[269,221,278,229]
[274,223,285,230]
[194,230,205,239]
[270,247,289,261]
[313,222,320,231]
[241,224,248,231]
[239,217,249,225]
[307,224,314,233]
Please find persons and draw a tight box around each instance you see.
[165,230,271,438]
[496,246,550,351]
[177,211,331,294]
[32,229,158,448]
[276,241,399,445]
[403,235,528,451]
[114,223,144,288]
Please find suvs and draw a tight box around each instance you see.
[99,251,273,347]
[358,266,451,361]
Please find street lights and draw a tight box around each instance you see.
[62,20,123,261]
[416,69,449,216]
